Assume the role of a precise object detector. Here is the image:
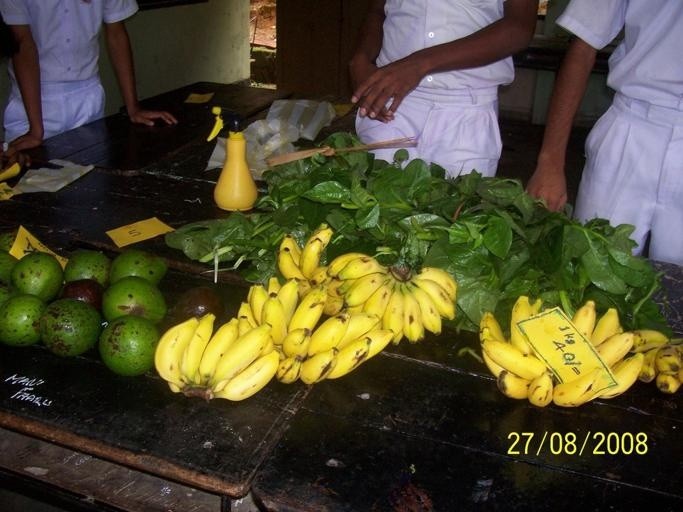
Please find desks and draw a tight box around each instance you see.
[0,74,680,505]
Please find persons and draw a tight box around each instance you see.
[526,0,683,267]
[348,0,539,180]
[0,0,179,157]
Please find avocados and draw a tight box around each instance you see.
[0,231,222,378]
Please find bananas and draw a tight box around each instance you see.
[154,220,458,401]
[477,295,683,408]
